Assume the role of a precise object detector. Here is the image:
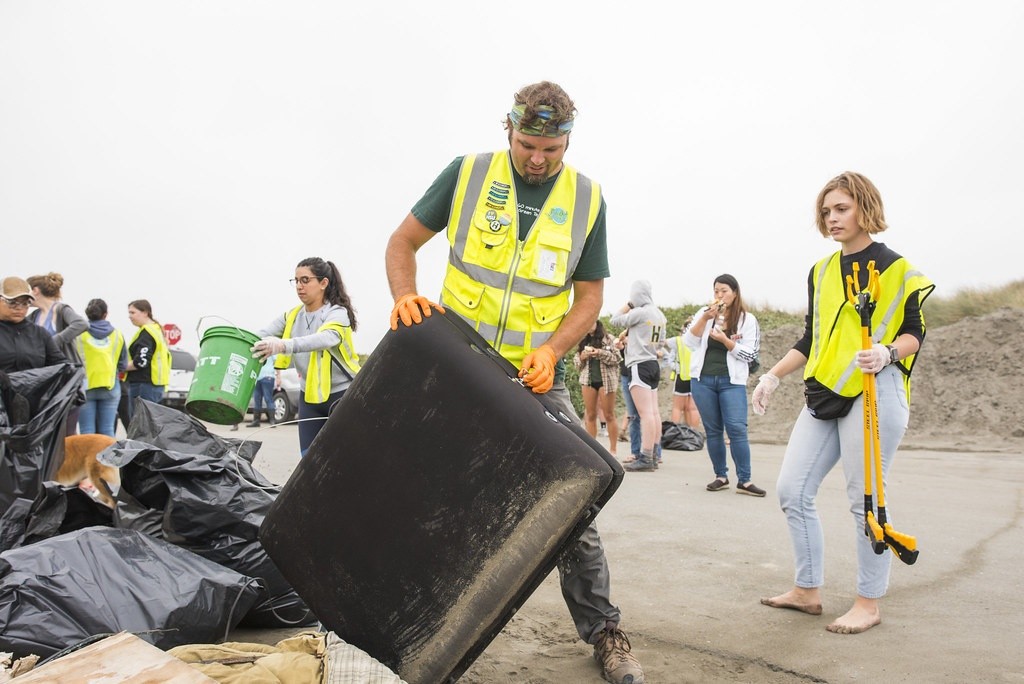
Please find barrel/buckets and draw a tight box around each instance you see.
[183,316,267,425]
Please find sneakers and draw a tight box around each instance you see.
[706,479,729,490]
[623,460,658,472]
[736,483,766,497]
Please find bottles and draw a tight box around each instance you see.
[712,316,725,336]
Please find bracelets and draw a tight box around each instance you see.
[628,302,635,308]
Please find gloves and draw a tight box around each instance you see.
[518,345,557,394]
[390,293,445,330]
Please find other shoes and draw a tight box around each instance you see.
[230,424,238,431]
[617,436,628,442]
[594,628,644,684]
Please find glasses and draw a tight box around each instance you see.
[1,297,32,307]
[289,276,320,286]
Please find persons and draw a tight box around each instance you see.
[384,81,647,684]
[683,274,766,498]
[574,278,700,472]
[252,256,363,460]
[245,353,277,428]
[0,271,173,438]
[748,170,936,634]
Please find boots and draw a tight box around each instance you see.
[246,409,261,427]
[265,409,276,427]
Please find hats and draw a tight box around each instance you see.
[0,276,35,300]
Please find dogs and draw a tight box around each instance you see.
[57,434,121,508]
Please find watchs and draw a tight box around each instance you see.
[886,345,899,364]
